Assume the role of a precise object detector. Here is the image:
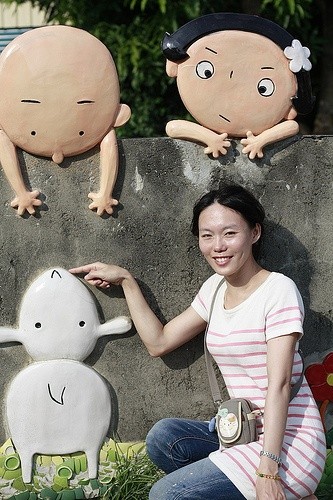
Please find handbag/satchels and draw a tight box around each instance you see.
[216,398,258,448]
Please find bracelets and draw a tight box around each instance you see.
[256,471,280,480]
[260,451,283,467]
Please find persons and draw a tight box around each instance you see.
[68,183,326,500]
